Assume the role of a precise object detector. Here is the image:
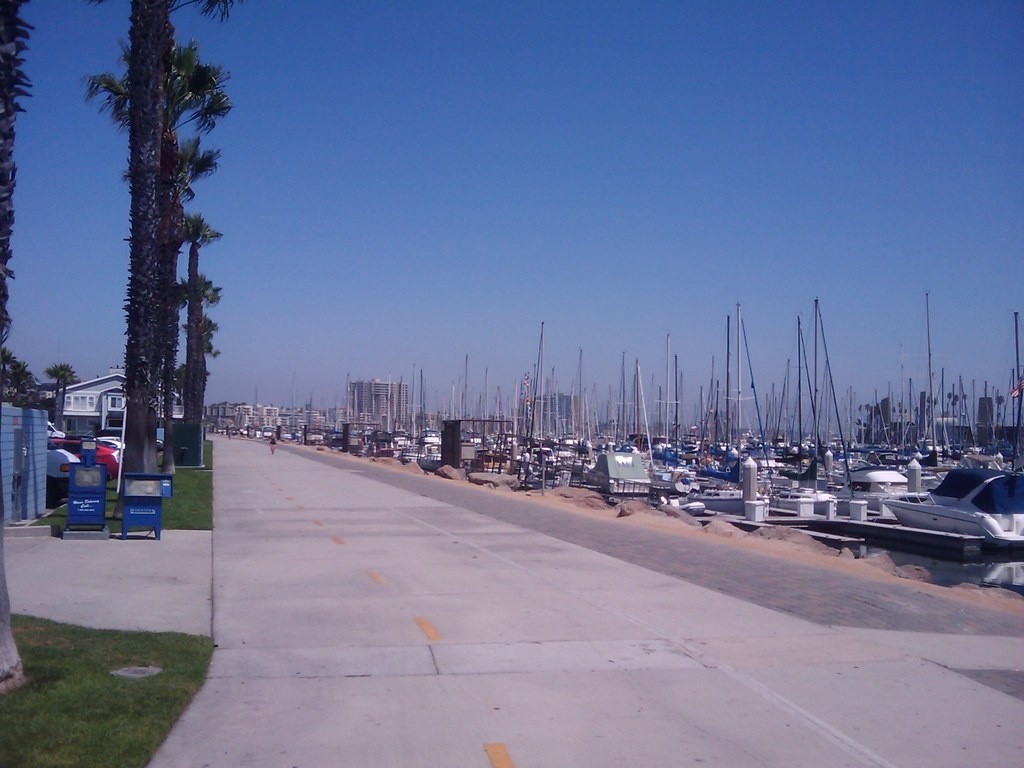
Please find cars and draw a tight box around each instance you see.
[47,419,164,504]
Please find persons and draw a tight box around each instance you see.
[228,429,231,438]
[270,432,276,454]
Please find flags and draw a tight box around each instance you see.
[691,426,697,432]
[1010,380,1024,398]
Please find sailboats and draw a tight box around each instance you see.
[290,286,1023,573]
